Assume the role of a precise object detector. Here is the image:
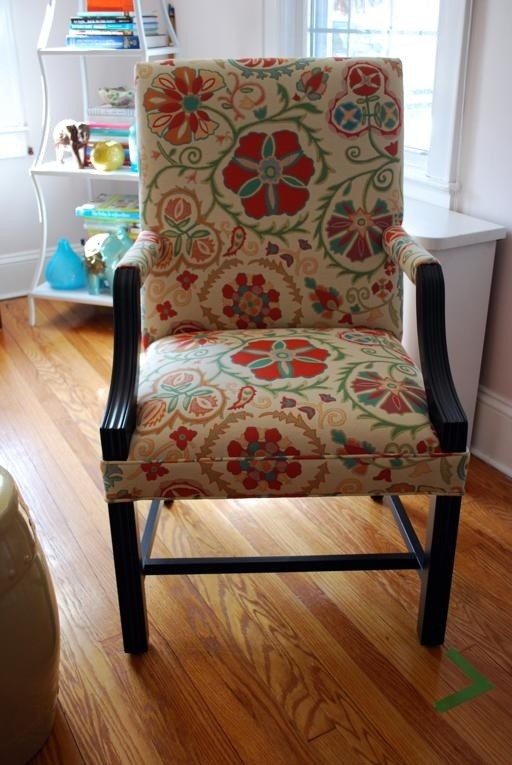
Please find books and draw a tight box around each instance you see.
[64,12,169,247]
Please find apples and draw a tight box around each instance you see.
[90,140,125,172]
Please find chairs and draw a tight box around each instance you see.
[101,51,477,654]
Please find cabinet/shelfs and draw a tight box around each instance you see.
[29,1,180,333]
